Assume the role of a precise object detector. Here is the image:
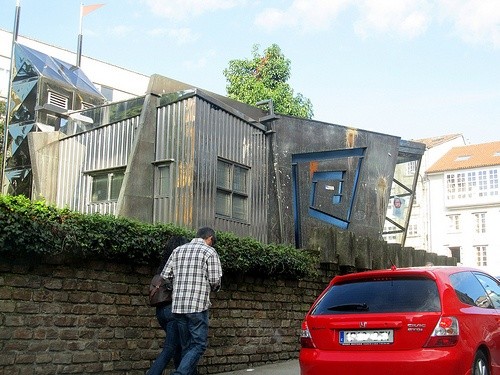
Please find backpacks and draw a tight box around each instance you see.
[149,273,173,307]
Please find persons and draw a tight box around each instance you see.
[161,227,223,375]
[145,272,182,375]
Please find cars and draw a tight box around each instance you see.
[299,264,500,375]
